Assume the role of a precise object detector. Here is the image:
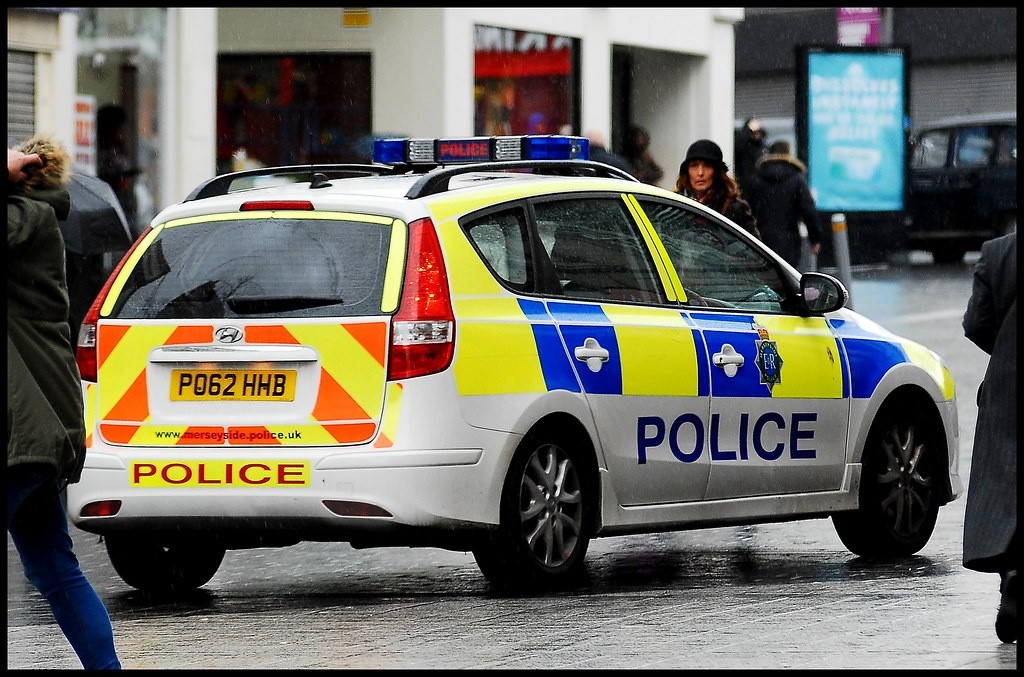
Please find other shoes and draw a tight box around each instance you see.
[995,569,1024,644]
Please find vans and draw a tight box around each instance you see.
[888,114,1018,264]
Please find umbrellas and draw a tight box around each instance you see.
[58,171,132,253]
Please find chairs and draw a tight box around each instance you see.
[553,225,642,296]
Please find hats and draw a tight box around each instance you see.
[685,139,723,164]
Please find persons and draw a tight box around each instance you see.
[960,229,1018,642]
[619,117,824,271]
[7,133,126,670]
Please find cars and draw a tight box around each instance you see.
[68,132,969,594]
